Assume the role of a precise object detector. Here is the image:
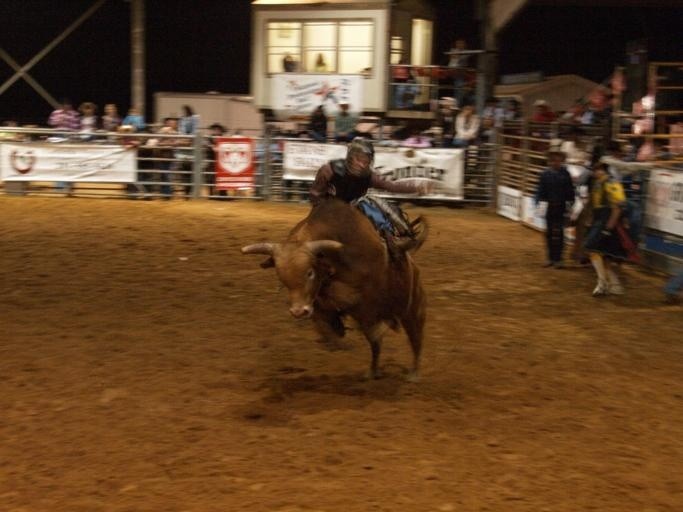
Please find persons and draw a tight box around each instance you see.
[310,137,434,257]
[528,44,656,168]
[257,101,378,200]
[578,164,628,297]
[0,121,40,194]
[154,105,229,201]
[48,101,146,196]
[534,148,574,269]
[389,96,521,149]
[284,55,300,72]
[313,53,327,72]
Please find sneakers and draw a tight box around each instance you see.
[592,280,625,296]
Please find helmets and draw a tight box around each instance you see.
[347,136,375,160]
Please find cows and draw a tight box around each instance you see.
[240,191,428,381]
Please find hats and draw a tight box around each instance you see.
[543,145,567,161]
